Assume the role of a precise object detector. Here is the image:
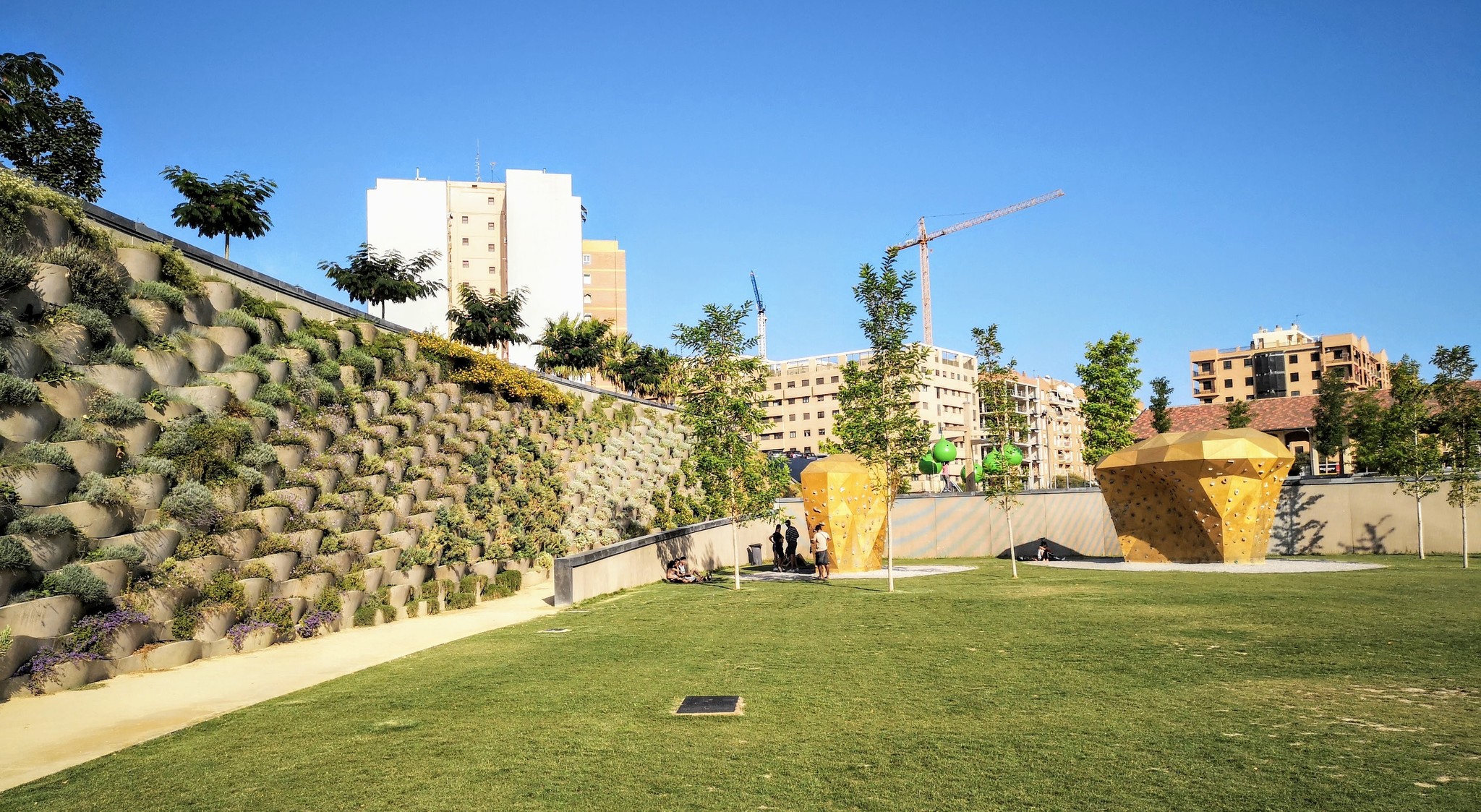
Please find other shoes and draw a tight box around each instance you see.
[1062,557,1066,560]
[1042,559,1049,562]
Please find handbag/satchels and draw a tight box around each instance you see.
[826,541,829,547]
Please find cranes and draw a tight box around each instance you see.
[750,269,768,358]
[884,189,1065,346]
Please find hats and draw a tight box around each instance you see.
[1041,541,1047,545]
[669,560,676,565]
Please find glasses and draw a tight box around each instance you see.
[677,562,681,564]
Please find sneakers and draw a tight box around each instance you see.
[703,573,713,582]
[773,566,799,573]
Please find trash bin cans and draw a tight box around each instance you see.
[748,543,762,566]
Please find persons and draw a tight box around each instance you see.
[1037,542,1066,562]
[769,520,799,573]
[789,452,797,459]
[667,556,713,584]
[942,462,951,492]
[811,525,832,580]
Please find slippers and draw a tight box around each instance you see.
[815,576,830,580]
